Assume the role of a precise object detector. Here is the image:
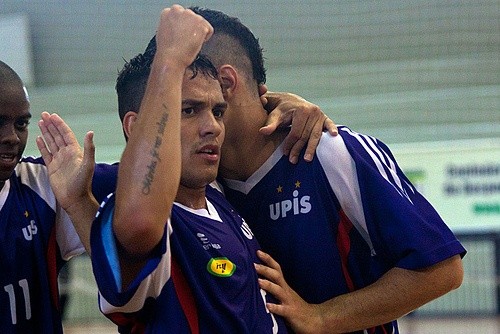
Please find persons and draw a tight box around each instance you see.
[88,3,339,334]
[0,61,267,334]
[37,7,466,333]
